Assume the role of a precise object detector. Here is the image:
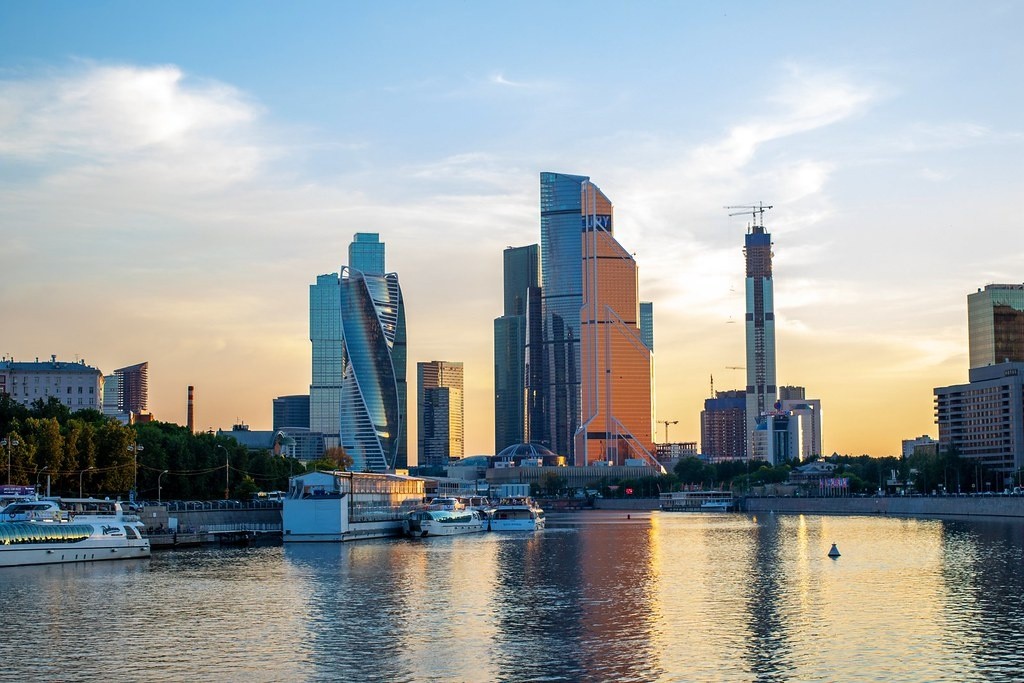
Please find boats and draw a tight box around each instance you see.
[0,501,152,567]
[659,490,733,513]
[489,495,547,531]
[400,493,491,538]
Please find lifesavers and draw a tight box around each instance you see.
[9,511,16,519]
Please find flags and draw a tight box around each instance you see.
[820,477,849,488]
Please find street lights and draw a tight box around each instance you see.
[1,434,20,487]
[80,466,94,498]
[158,470,169,506]
[127,441,145,500]
[36,466,49,495]
[218,445,229,500]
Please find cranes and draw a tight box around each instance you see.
[724,200,774,229]
[657,419,679,444]
